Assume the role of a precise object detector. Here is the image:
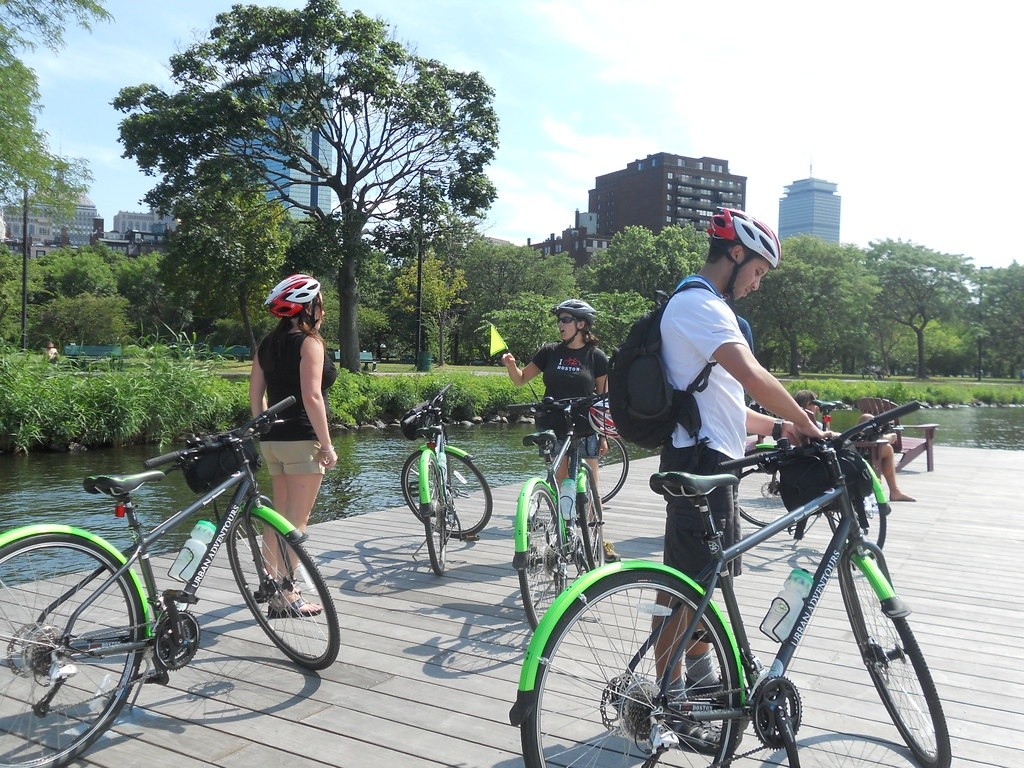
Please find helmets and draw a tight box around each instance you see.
[588,397,621,440]
[707,207,780,268]
[262,274,322,318]
[553,298,595,332]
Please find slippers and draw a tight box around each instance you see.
[255,584,279,604]
[268,597,317,618]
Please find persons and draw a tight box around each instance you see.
[793,389,919,502]
[46,342,60,365]
[501,299,621,563]
[649,206,838,756]
[248,274,338,619]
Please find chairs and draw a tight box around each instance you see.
[800,397,940,482]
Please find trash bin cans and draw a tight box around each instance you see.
[417,351,432,372]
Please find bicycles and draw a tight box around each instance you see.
[508,397,954,768]
[507,392,630,633]
[1,395,341,768]
[400,382,493,577]
[738,399,891,560]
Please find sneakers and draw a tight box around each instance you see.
[594,541,620,562]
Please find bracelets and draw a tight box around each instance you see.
[772,419,784,442]
[319,447,335,454]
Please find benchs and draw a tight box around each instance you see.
[213,347,252,362]
[168,343,207,361]
[381,356,396,360]
[334,349,381,372]
[862,367,881,380]
[64,345,128,371]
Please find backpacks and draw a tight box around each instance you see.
[607,281,729,449]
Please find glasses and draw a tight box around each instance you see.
[556,316,577,324]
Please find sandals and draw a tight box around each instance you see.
[668,717,723,755]
[686,677,730,708]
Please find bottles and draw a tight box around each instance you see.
[439,452,448,484]
[560,479,576,520]
[169,520,217,582]
[762,568,815,643]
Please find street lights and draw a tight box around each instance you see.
[413,167,438,364]
